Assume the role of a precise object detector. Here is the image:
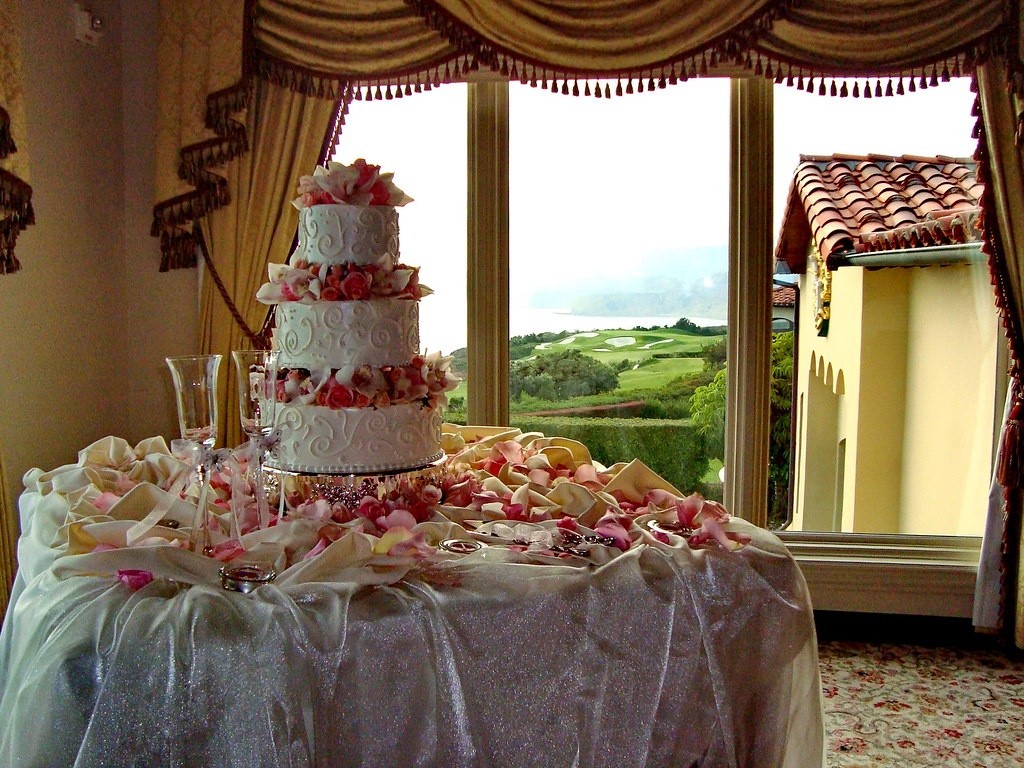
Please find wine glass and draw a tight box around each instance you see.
[230,350,285,534]
[165,353,223,557]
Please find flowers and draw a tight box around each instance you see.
[87,157,755,599]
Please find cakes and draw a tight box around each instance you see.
[250,158,443,473]
